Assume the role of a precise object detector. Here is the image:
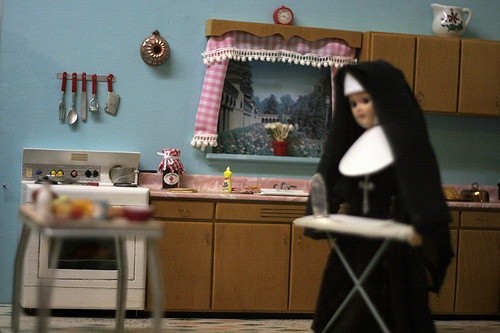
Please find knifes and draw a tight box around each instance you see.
[104,74,120,115]
[82,72,86,120]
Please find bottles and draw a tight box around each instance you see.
[162,149,179,188]
[222,167,232,192]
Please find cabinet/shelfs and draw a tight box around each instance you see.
[357,31,500,117]
[428,208,500,321]
[144,197,336,319]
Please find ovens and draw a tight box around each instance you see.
[18,204,148,315]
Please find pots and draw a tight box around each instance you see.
[22,147,149,205]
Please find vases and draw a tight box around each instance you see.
[272,142,289,155]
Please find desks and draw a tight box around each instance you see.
[11,205,162,333]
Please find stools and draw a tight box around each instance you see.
[293,215,413,333]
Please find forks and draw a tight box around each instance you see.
[58,72,67,120]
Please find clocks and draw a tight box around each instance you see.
[273,5,293,25]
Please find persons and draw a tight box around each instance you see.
[302,59,455,333]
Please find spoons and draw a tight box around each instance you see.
[67,73,77,125]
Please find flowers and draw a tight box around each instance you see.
[265,122,298,142]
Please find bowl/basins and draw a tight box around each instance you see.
[112,167,140,187]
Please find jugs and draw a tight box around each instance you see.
[432,4,472,35]
